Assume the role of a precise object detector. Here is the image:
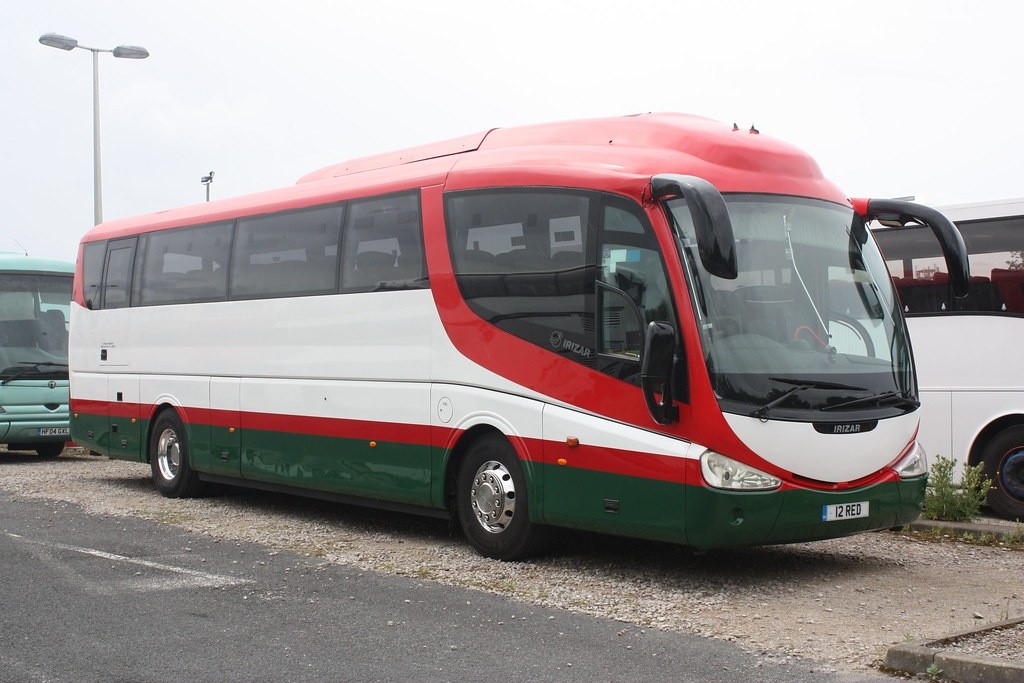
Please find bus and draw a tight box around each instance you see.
[669,188,1024,527]
[0,249,75,458]
[64,107,972,564]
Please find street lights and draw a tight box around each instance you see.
[200,171,216,202]
[37,31,151,226]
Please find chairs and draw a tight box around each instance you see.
[46,309,64,330]
[775,268,1024,312]
[157,248,584,298]
